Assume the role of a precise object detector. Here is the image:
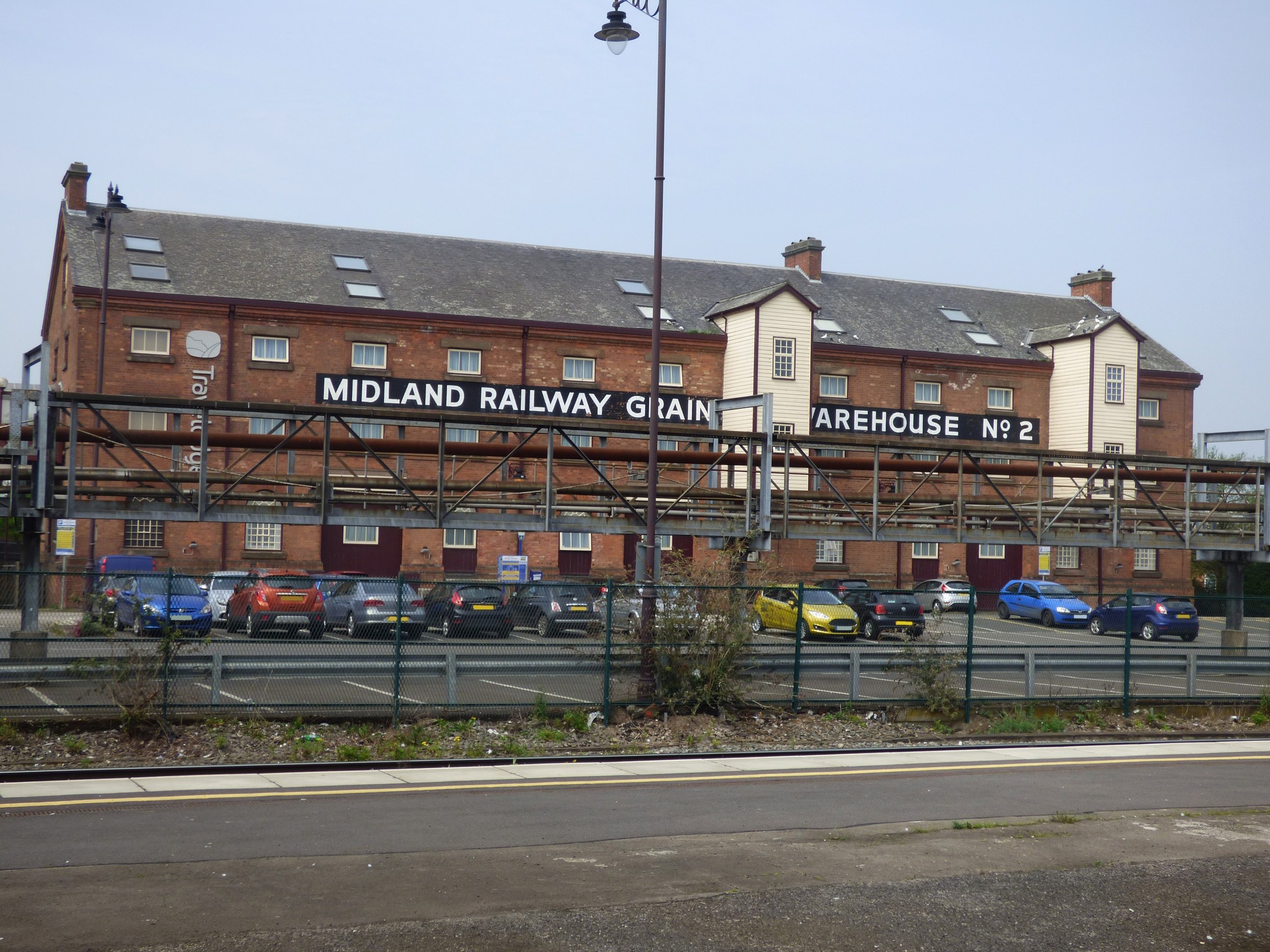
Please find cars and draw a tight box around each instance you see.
[90,572,139,628]
[423,581,513,639]
[596,580,703,639]
[114,576,213,639]
[196,570,251,623]
[751,584,860,641]
[1088,592,1199,642]
[912,579,978,614]
[505,579,603,638]
[325,570,369,578]
[996,579,1093,629]
[322,579,428,640]
[311,574,353,601]
[813,578,870,601]
[840,592,926,640]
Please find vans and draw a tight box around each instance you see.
[91,555,156,591]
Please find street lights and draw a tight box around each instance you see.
[593,0,668,700]
[84,177,132,628]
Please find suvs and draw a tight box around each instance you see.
[226,567,327,641]
